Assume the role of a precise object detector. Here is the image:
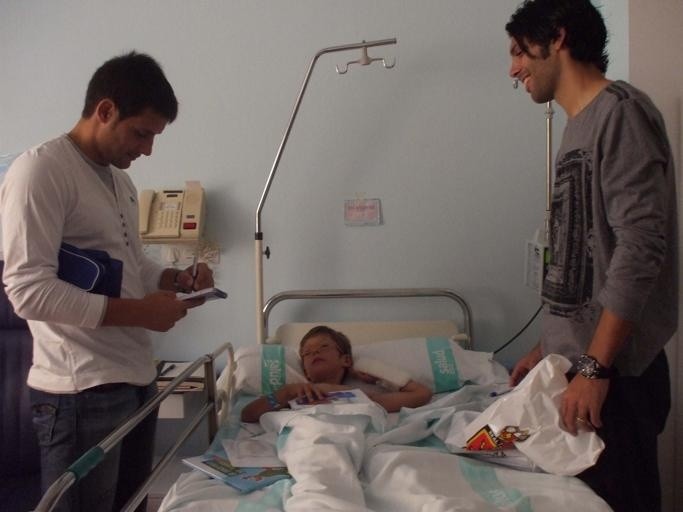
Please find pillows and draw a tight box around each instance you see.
[237,339,464,398]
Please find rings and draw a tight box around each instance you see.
[174,270,183,283]
[575,416,586,424]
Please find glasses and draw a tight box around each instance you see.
[301,340,345,357]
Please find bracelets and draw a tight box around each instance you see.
[265,393,280,410]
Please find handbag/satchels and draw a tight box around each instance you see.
[56,241,123,297]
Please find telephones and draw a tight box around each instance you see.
[138,188,206,242]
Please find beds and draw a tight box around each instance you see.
[31,287,611,512]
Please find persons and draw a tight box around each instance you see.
[238,324,434,425]
[0,49,218,510]
[501,1,683,511]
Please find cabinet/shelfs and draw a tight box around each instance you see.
[147,385,210,499]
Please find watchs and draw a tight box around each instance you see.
[572,353,611,383]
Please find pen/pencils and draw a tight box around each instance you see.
[161,362,175,375]
[192,257,197,293]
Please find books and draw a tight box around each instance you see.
[180,450,292,495]
[154,360,206,393]
[174,286,226,307]
[288,386,376,412]
